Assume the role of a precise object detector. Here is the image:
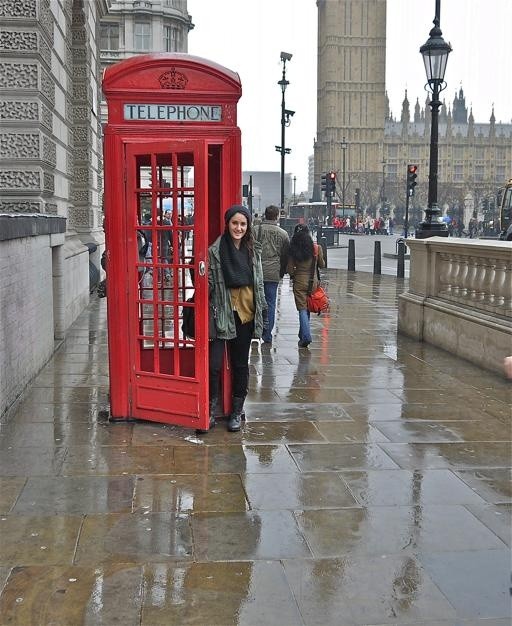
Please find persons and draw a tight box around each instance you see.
[448,215,499,239]
[95,206,195,299]
[173,439,265,626]
[284,222,327,351]
[242,354,283,467]
[278,351,332,461]
[250,204,290,346]
[312,213,396,237]
[186,205,268,437]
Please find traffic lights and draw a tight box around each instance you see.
[327,171,337,192]
[320,173,327,191]
[148,170,158,187]
[406,163,418,194]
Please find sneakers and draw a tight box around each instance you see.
[297,333,312,348]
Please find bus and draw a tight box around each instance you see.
[288,200,360,227]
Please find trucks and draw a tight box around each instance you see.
[496,177,512,232]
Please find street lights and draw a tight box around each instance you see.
[274,67,291,224]
[340,134,348,217]
[380,154,388,217]
[415,1,455,236]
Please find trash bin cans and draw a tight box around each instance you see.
[322,227,335,246]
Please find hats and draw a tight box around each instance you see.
[224,205,252,228]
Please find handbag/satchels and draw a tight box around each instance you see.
[306,243,328,315]
[179,297,217,342]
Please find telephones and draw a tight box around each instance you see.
[137,230,149,272]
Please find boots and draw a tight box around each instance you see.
[197,397,219,434]
[227,395,246,432]
